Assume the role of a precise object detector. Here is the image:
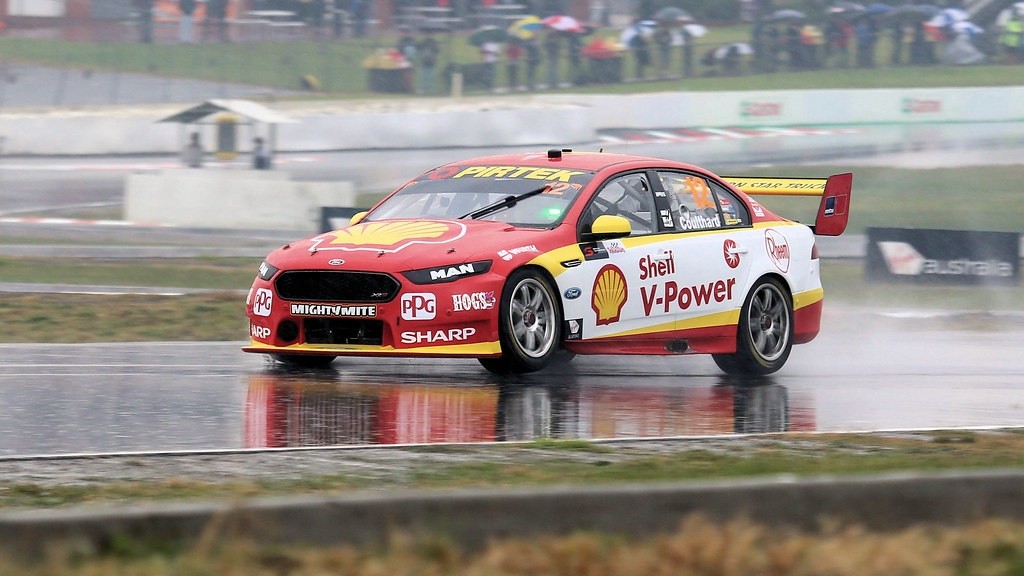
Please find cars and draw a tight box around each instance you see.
[240,148,853,378]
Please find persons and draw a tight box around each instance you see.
[179,0,230,44]
[183,132,203,167]
[254,138,271,170]
[396,12,944,94]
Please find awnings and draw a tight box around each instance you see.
[154,98,301,158]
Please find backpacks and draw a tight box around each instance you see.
[423,51,435,66]
[405,47,417,61]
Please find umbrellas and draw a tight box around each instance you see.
[400,0,985,64]
